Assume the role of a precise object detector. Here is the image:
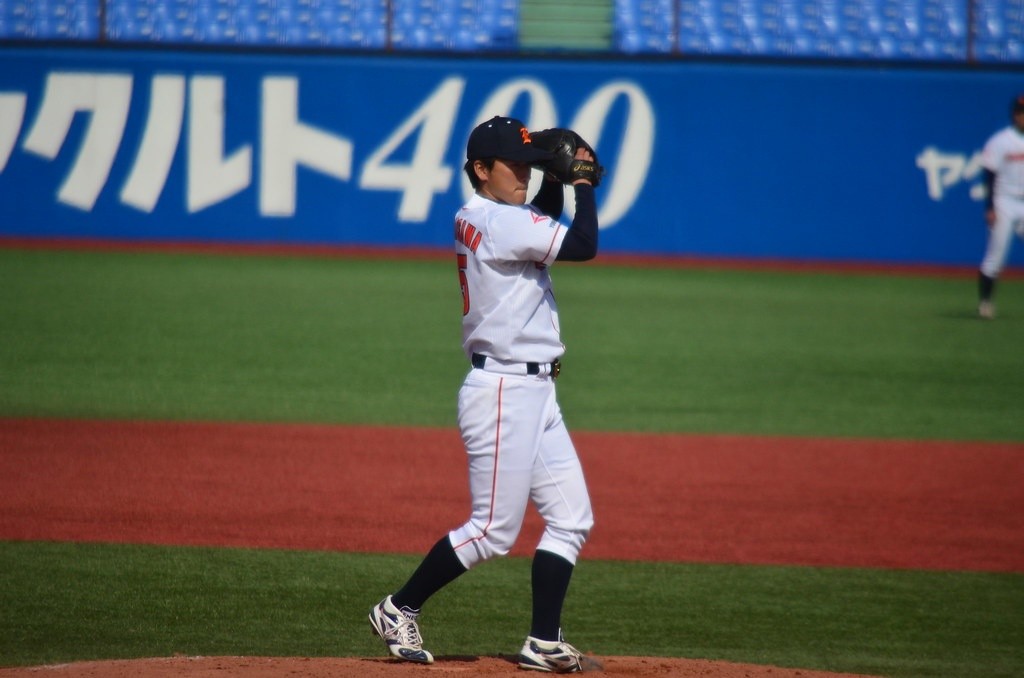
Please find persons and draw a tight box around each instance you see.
[976,96,1024,319]
[368,115,602,674]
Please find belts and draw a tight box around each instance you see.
[472,353,561,378]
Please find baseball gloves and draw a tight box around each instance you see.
[529,129,605,189]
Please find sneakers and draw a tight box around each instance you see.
[367,594,434,664]
[518,627,603,673]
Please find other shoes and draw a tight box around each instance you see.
[976,302,993,320]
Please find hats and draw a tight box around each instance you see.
[467,115,562,161]
[1013,96,1024,111]
